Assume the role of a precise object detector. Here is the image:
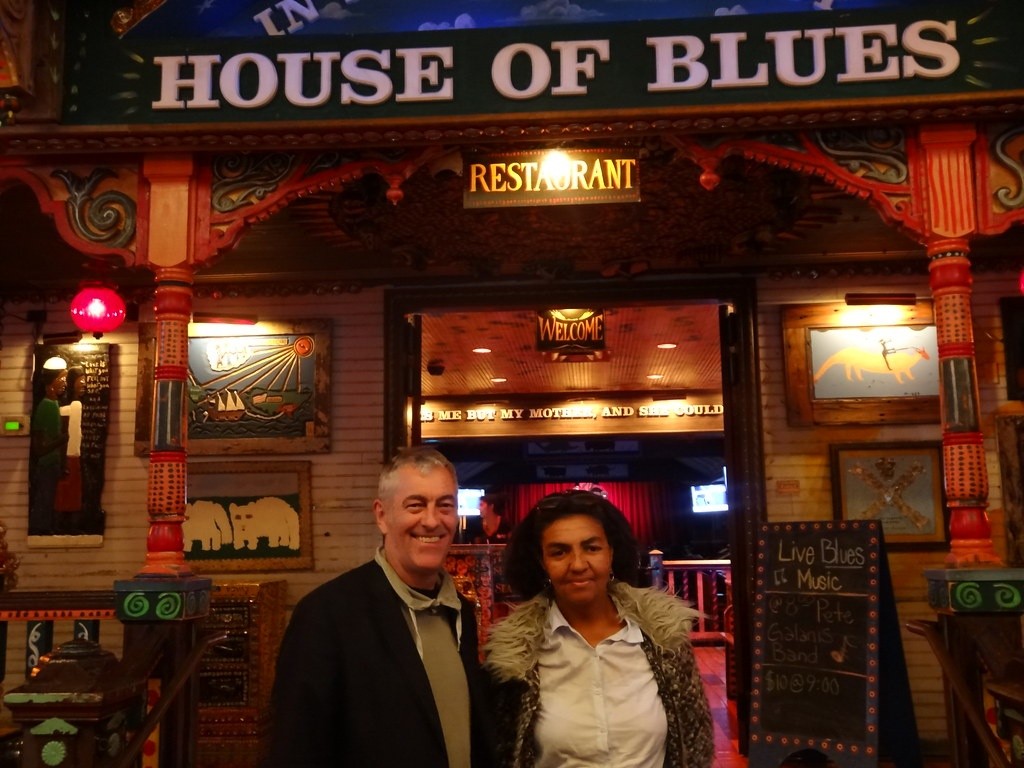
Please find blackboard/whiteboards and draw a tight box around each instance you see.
[747,520,918,750]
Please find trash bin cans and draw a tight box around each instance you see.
[2,639,148,768]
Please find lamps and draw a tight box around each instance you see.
[69,255,125,340]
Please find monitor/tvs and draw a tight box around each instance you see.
[690,485,729,513]
[457,489,485,516]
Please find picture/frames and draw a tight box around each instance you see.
[997,416,1024,568]
[182,461,314,571]
[829,440,949,549]
[135,320,331,457]
[783,298,941,427]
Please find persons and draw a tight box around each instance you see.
[483,490,715,768]
[256,448,526,768]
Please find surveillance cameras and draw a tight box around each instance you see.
[427,361,447,376]
